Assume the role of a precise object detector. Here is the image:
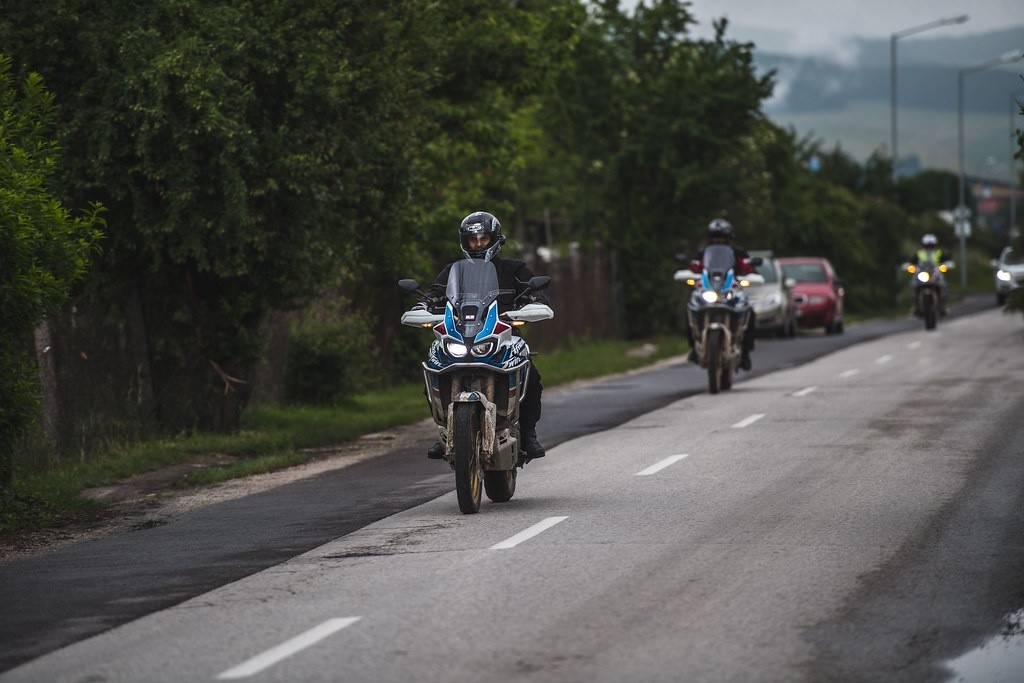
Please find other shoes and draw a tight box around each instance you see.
[740,352,752,370]
[688,349,698,361]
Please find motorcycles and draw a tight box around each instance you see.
[901,260,955,330]
[398,259,554,513]
[672,248,764,393]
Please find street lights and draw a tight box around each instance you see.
[888,13,969,201]
[1008,84,1024,237]
[960,51,1024,288]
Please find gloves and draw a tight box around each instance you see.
[411,303,428,311]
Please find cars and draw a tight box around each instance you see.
[988,247,1024,307]
[733,256,796,338]
[776,257,845,334]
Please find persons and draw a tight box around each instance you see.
[913,234,955,319]
[687,219,759,372]
[411,211,550,459]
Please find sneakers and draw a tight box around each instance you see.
[428,442,445,459]
[520,422,545,458]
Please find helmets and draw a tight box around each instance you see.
[707,219,733,246]
[921,233,938,245]
[459,211,507,264]
